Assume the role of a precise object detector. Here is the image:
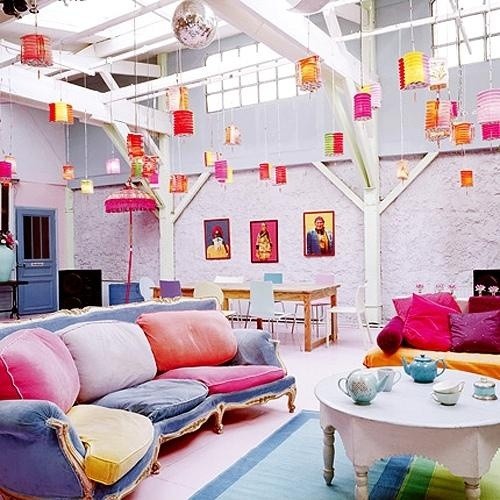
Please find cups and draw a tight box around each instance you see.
[430,379,467,407]
[377,367,402,393]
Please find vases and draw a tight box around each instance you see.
[0,244,13,282]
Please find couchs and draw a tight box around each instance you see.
[364,299,500,379]
[0,295,298,500]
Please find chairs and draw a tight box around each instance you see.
[139,273,373,352]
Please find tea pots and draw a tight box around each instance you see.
[337,367,389,407]
[400,352,446,383]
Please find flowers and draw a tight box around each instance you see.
[0,230,19,249]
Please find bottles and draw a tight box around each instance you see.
[470,376,497,400]
[9,268,15,281]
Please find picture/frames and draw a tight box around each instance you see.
[303,211,335,257]
[249,219,279,263]
[203,218,231,260]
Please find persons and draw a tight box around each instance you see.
[207,227,230,259]
[256,223,272,260]
[307,216,333,254]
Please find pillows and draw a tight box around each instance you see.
[392,293,500,354]
[0,310,238,415]
